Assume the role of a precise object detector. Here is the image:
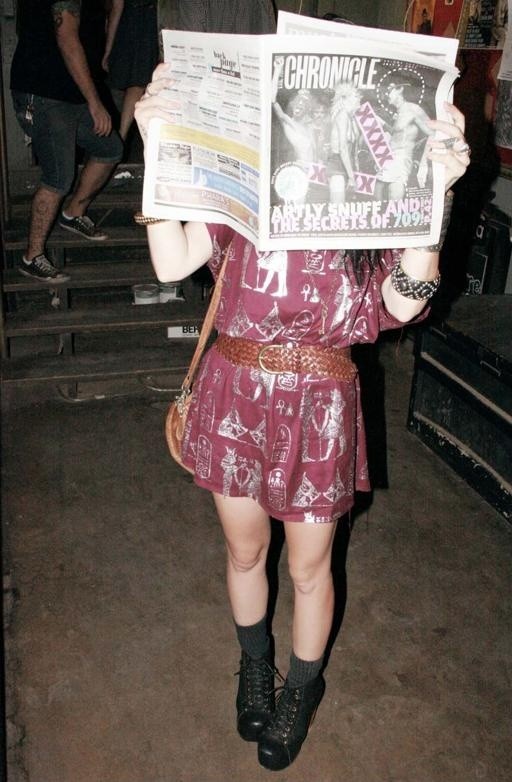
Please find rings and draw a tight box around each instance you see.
[456,143,472,158]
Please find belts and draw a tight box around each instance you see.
[216,332,358,382]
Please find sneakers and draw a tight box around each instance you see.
[19,253,71,282]
[58,211,108,241]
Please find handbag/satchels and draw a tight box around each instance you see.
[165,392,194,475]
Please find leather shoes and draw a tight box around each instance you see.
[234,634,325,770]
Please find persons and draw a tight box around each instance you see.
[415,9,433,38]
[167,1,279,35]
[100,2,162,163]
[7,0,126,286]
[372,76,434,203]
[268,85,325,217]
[321,82,364,206]
[129,60,474,776]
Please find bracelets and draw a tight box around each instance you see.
[416,188,458,253]
[133,209,177,229]
[389,263,442,301]
